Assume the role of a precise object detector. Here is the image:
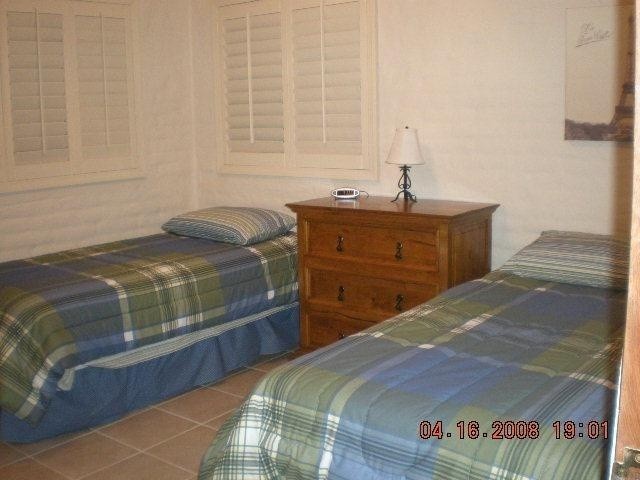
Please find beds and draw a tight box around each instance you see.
[194,269,629,480]
[0,232,304,443]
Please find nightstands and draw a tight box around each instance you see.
[284,195,500,351]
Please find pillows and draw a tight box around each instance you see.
[161,205,296,247]
[501,229,630,293]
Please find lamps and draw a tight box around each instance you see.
[385,124,423,204]
